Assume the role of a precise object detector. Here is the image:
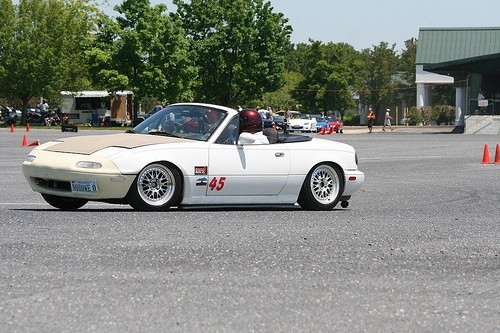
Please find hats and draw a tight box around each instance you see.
[386,109,390,112]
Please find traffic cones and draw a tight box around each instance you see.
[25,123,32,132]
[11,124,15,134]
[21,134,29,147]
[28,140,40,146]
[480,144,492,164]
[493,144,500,164]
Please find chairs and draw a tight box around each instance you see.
[230,124,238,138]
[263,128,280,144]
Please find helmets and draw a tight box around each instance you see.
[239,110,262,132]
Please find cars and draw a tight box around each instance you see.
[0,106,60,124]
[301,114,329,133]
[326,117,343,133]
[287,113,316,133]
[263,113,287,132]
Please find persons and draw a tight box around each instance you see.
[382,108,394,132]
[4,108,18,127]
[234,109,270,145]
[39,99,50,126]
[169,110,175,125]
[366,107,375,133]
[198,108,233,145]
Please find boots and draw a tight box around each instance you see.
[340,130,343,133]
[336,130,339,133]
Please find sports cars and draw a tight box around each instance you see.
[21,102,365,212]
